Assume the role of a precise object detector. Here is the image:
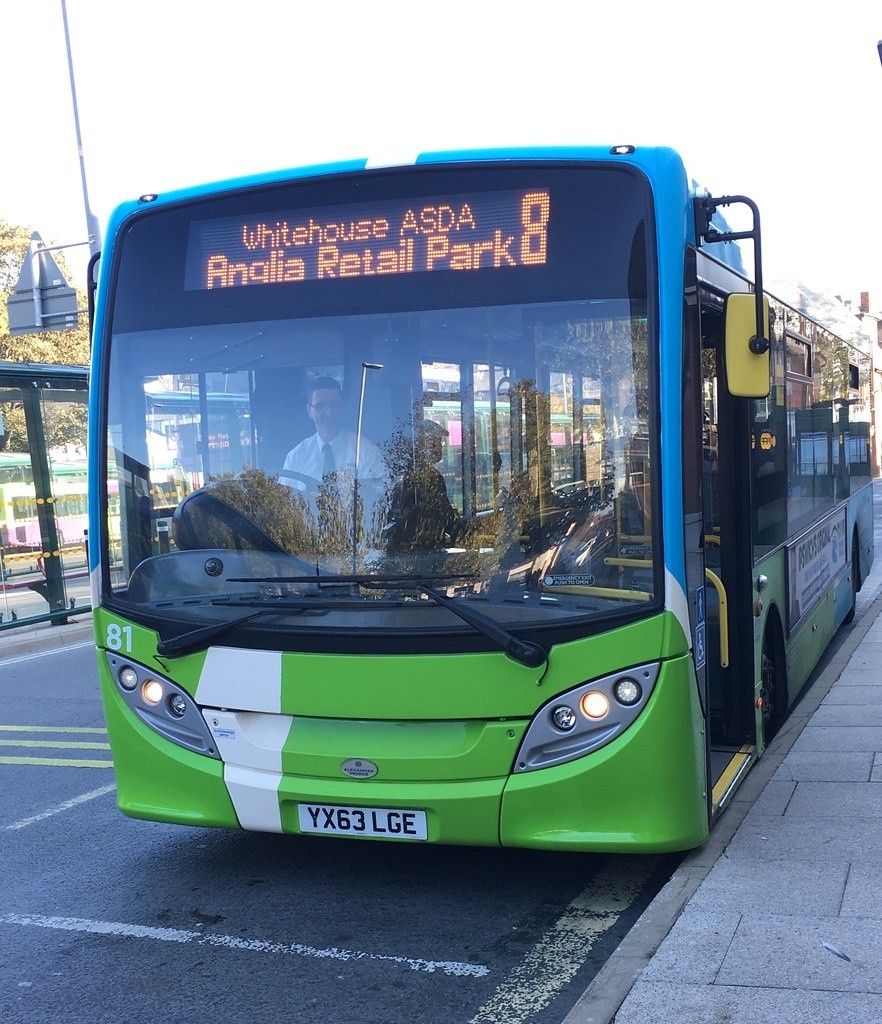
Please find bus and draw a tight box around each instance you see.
[83,144,876,855]
[1,450,206,559]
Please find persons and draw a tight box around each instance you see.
[277,376,390,553]
[389,419,459,551]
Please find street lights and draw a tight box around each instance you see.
[352,363,383,578]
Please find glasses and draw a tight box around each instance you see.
[310,404,345,412]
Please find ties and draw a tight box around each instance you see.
[319,445,338,540]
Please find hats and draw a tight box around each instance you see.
[422,419,449,437]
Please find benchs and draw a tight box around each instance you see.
[1,563,124,616]
